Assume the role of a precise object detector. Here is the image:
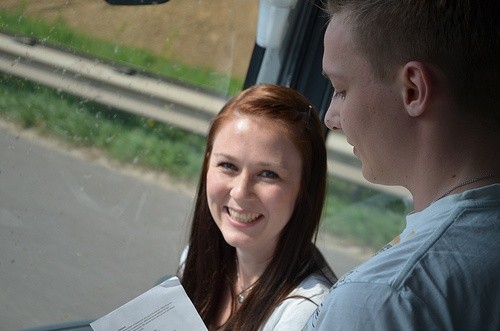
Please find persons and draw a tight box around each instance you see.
[295,0,500,330]
[174,82,340,331]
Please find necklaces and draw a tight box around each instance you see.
[424,162,500,209]
[234,256,259,305]
